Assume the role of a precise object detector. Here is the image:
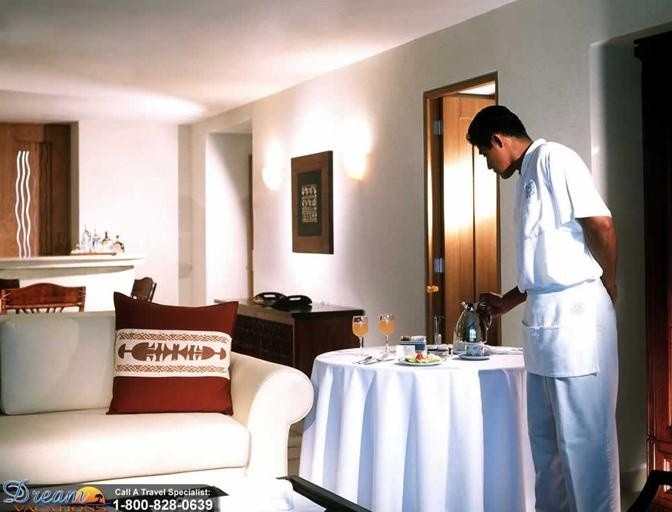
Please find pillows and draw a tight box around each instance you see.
[109,289,239,415]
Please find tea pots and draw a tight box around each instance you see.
[455,301,492,344]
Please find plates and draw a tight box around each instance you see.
[459,353,489,362]
[400,354,447,367]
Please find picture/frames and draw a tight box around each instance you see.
[289,152,334,252]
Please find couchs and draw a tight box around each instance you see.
[0,312,315,487]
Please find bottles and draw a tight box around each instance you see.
[76,225,125,253]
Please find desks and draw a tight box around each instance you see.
[213,298,364,380]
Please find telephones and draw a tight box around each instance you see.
[271,294,313,309]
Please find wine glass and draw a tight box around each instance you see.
[377,313,394,358]
[351,316,369,357]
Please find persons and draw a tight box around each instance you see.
[465,104,619,512]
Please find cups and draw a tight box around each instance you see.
[464,342,490,357]
[452,331,466,354]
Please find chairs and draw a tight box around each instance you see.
[0,277,155,314]
